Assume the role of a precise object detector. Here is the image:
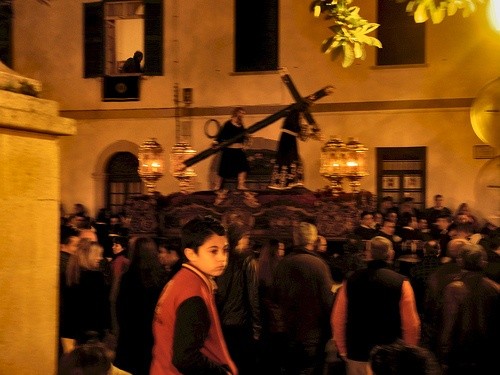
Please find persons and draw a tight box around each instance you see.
[212,107,249,190]
[268,102,314,191]
[213,195,500,375]
[122,51,143,73]
[150,215,238,375]
[59,202,185,375]
